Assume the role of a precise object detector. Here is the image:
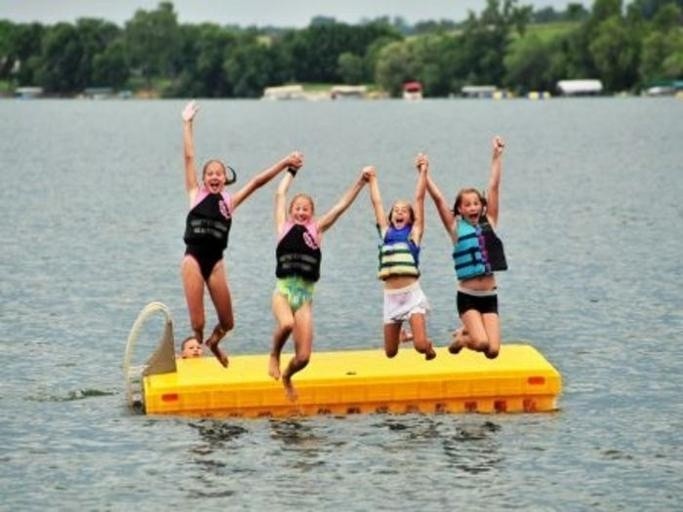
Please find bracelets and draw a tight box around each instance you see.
[286,166,297,178]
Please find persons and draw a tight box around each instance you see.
[413,135,507,362]
[364,153,437,363]
[173,337,209,359]
[266,151,373,406]
[177,97,304,370]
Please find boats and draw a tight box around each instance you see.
[116,300,568,423]
